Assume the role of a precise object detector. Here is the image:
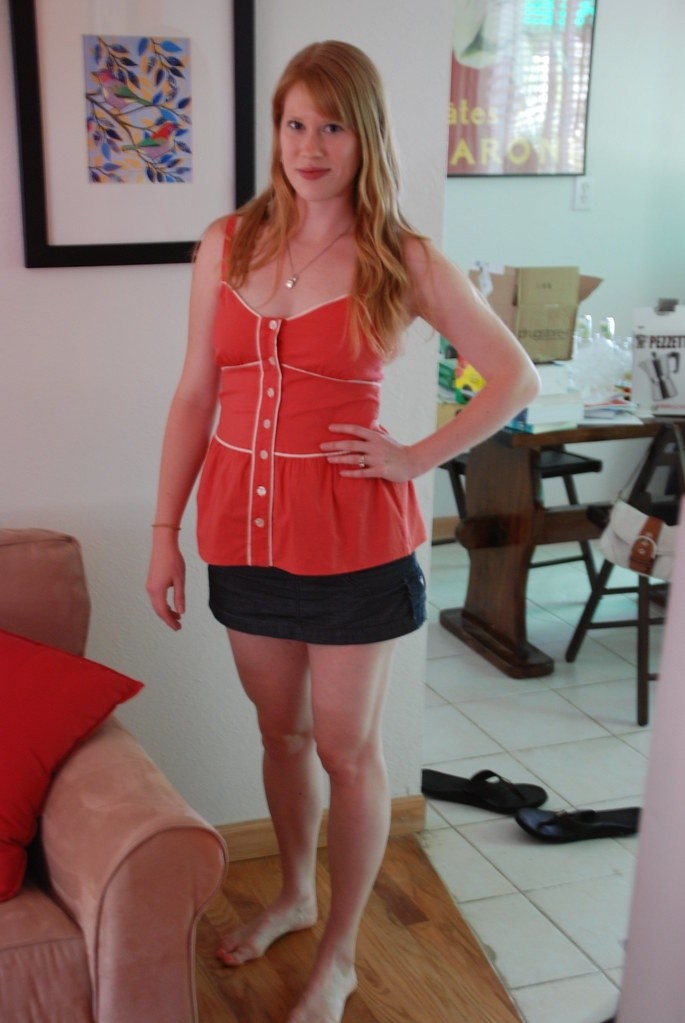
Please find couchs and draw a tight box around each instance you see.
[0,527,225,1021]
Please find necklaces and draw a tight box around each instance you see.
[278,213,357,289]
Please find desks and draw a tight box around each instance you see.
[440,404,685,678]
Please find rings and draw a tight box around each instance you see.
[358,455,367,470]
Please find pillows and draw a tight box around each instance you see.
[1,629,145,902]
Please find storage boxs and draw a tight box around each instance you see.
[471,265,604,363]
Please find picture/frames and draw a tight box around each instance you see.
[9,0,257,268]
[449,1,600,179]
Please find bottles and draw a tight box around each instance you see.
[597,314,616,342]
[576,313,592,354]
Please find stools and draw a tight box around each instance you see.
[438,444,603,598]
[565,419,685,726]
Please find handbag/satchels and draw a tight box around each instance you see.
[598,426,685,583]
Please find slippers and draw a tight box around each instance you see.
[421,765,548,815]
[514,804,641,842]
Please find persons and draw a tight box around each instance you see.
[141,38,545,1023]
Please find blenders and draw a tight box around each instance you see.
[637,348,679,401]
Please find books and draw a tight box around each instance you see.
[455,351,647,434]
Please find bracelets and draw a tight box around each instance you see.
[149,521,180,531]
